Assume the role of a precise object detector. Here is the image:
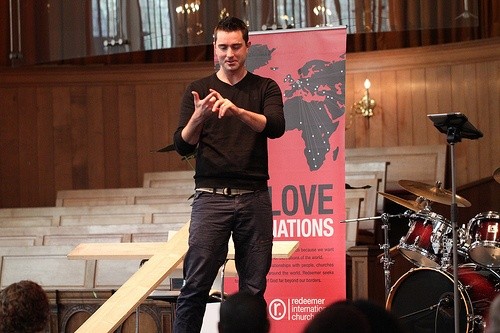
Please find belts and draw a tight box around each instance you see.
[198,185,268,197]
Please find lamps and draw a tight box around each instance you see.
[357,79,376,116]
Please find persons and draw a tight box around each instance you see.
[0,281,50,333]
[218,291,270,333]
[303,299,407,333]
[173,17,286,333]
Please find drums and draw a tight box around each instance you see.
[385,262,500,333]
[389,248,445,291]
[466,208,500,267]
[397,209,458,269]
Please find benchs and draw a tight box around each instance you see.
[0,160,390,293]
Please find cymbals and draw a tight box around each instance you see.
[397,178,472,209]
[492,167,500,186]
[375,189,423,212]
